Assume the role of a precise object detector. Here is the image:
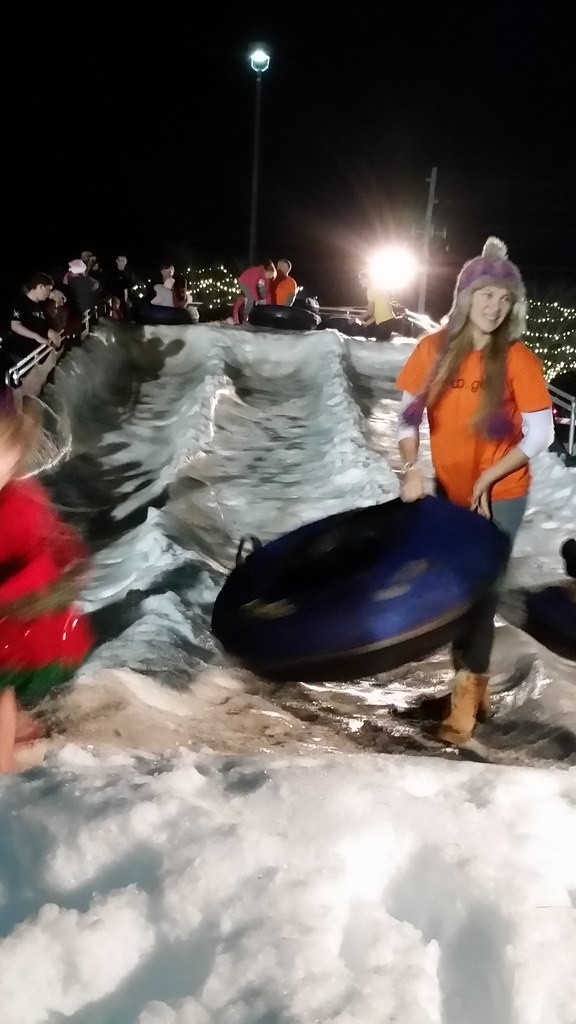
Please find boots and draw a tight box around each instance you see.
[438,670,491,745]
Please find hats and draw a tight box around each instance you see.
[400,235,522,442]
[68,260,87,274]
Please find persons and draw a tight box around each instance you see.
[391,236,556,745]
[1,400,93,779]
[11,250,200,354]
[233,259,298,327]
[349,270,396,342]
